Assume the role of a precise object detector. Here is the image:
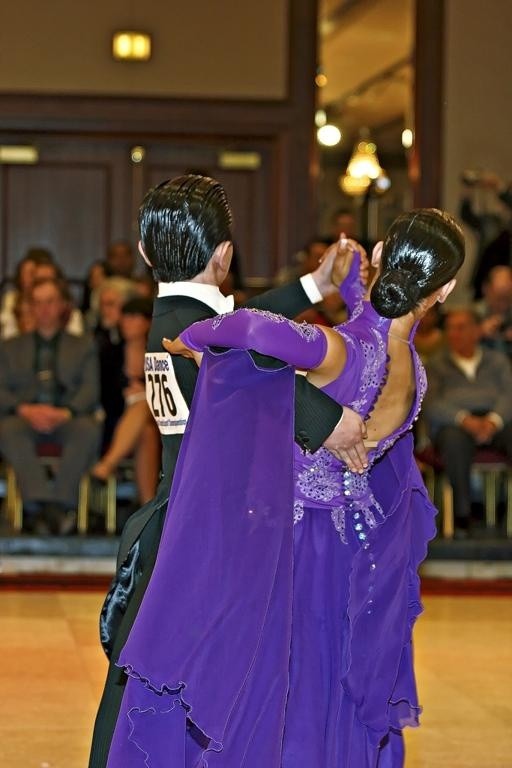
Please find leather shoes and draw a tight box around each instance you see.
[89,467,108,487]
[32,509,76,538]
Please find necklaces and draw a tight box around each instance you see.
[389,333,411,346]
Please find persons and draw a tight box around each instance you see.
[0,169,512,538]
[83,168,371,765]
[106,206,466,765]
